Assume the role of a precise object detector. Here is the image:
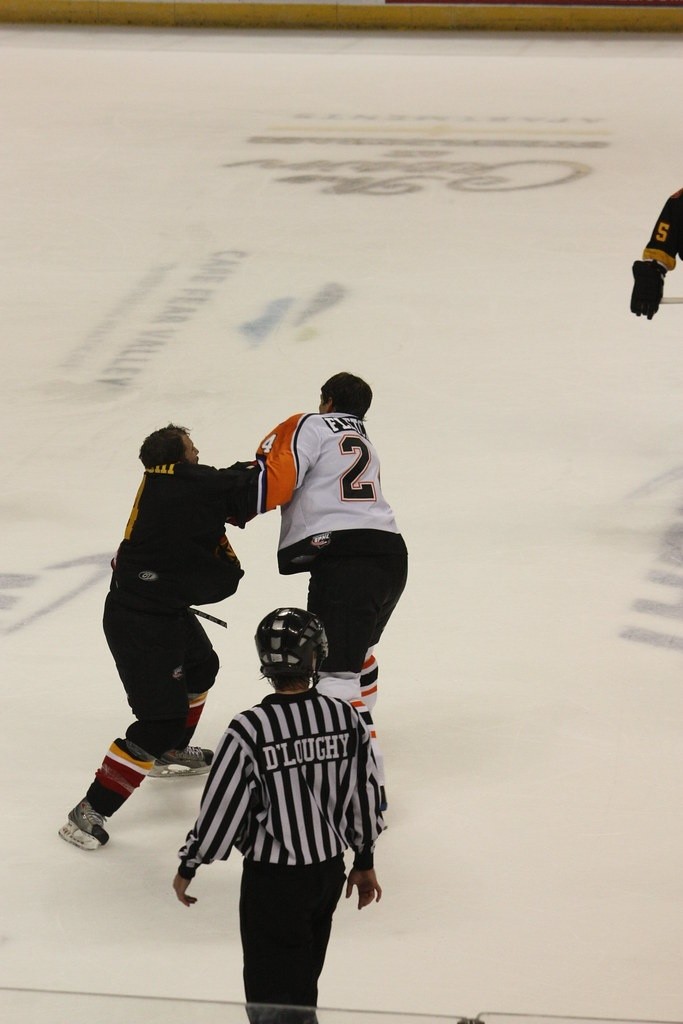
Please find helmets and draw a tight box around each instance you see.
[252,607,328,691]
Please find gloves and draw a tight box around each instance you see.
[630,259,668,319]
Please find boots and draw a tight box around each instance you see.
[146,745,214,778]
[57,796,108,851]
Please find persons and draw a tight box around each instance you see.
[229,372,409,830]
[630,188,683,319]
[172,607,388,1024]
[59,426,259,850]
